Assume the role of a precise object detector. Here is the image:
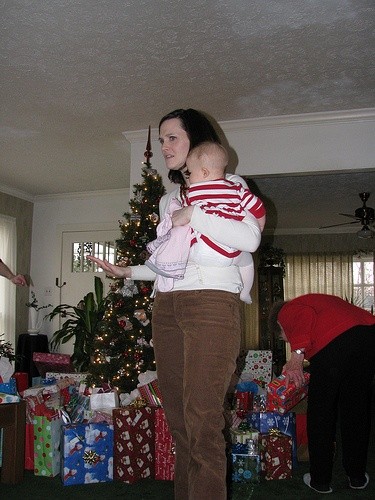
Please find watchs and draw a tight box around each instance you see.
[294,349,305,355]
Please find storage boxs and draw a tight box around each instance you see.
[21,350,310,486]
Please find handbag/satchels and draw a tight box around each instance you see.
[61,421,114,486]
[32,411,60,478]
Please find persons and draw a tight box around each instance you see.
[87,107,261,500]
[266,294,375,494]
[0,259,27,288]
[178,141,266,304]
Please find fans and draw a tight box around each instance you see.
[320,192,375,229]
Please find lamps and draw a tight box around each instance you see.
[356,225,375,239]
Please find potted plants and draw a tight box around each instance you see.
[256,244,286,279]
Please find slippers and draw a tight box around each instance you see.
[348,471,370,489]
[303,471,333,494]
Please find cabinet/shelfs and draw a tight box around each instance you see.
[257,266,286,377]
